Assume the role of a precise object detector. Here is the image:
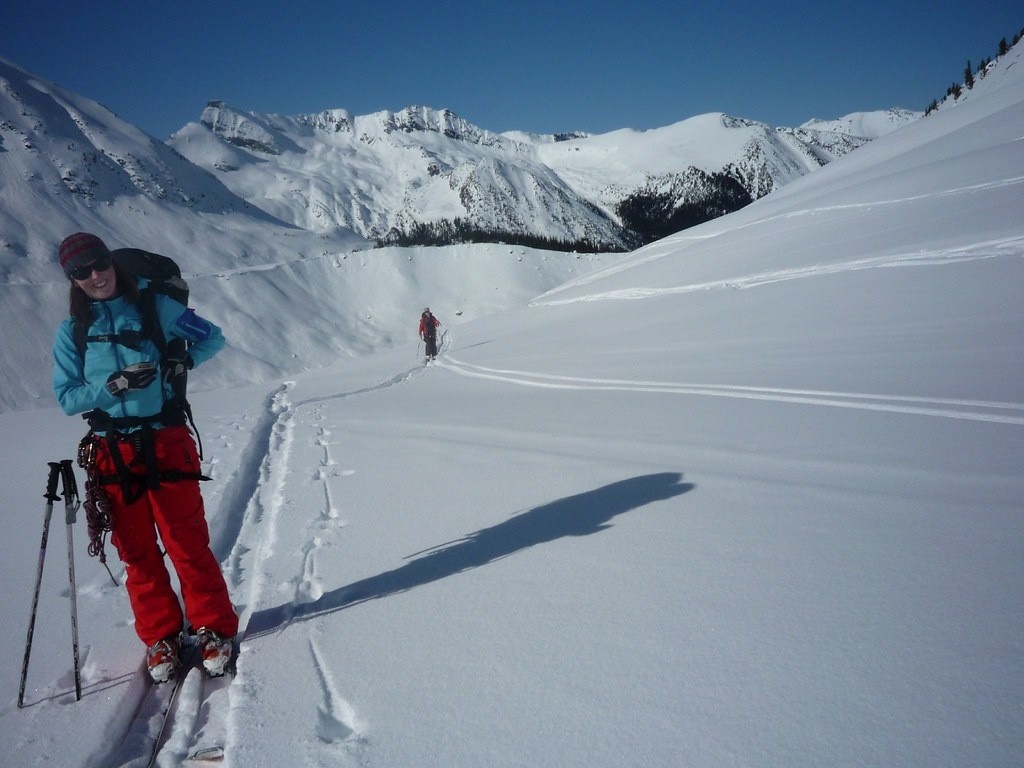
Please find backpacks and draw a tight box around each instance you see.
[73,247,189,363]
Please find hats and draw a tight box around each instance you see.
[59,232,108,273]
[424,308,429,312]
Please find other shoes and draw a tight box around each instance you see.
[432,357,436,360]
[426,355,430,359]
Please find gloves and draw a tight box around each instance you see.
[107,362,157,395]
[161,351,195,390]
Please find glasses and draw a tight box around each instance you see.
[69,255,110,280]
[425,311,429,313]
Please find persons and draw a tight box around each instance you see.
[418,307,439,361]
[51,232,239,681]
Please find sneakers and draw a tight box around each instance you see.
[194,627,235,677]
[146,631,184,683]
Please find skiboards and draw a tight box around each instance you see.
[103,604,239,768]
